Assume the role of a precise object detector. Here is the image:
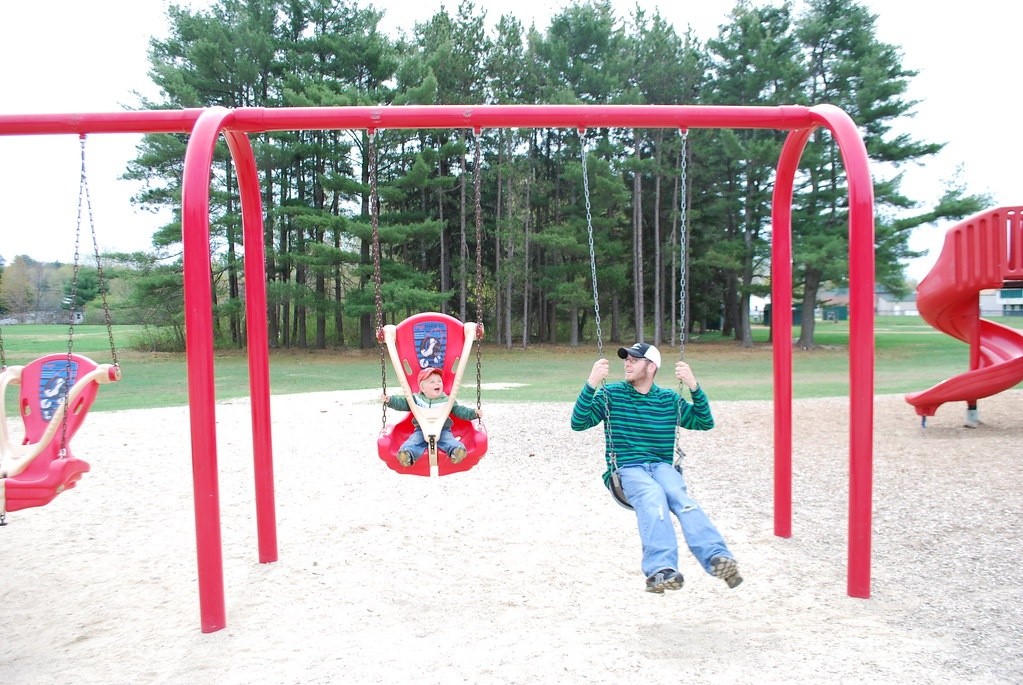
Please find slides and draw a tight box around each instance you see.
[905,285,1023,418]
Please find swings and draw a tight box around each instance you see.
[367,136,490,477]
[0,140,123,529]
[580,139,692,512]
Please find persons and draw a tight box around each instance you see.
[378,367,483,467]
[571,343,743,592]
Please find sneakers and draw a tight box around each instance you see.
[710,555,743,588]
[645,569,684,594]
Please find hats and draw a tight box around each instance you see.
[617,342,661,369]
[417,368,443,387]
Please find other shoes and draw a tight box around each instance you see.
[450,447,467,463]
[396,449,413,467]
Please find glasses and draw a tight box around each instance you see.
[623,357,652,363]
